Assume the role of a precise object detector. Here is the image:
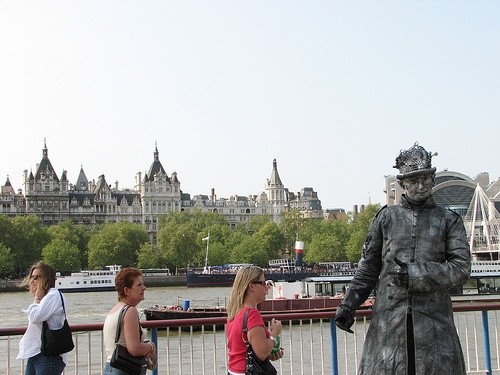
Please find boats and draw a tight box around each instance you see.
[186,229,328,288]
[141,279,372,333]
[52,264,123,293]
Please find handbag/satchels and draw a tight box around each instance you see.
[109,304,148,375]
[40,292,75,356]
[242,310,277,375]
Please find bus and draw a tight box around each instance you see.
[226,263,254,274]
[138,267,169,277]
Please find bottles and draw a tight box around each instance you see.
[272,335,280,354]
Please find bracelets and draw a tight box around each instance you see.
[270,336,278,345]
[149,342,154,351]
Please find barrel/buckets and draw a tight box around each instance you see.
[183,301,189,311]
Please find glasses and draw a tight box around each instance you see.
[31,275,42,280]
[253,280,267,286]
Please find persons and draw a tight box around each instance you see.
[479,283,500,295]
[226,266,284,375]
[335,142,472,375]
[103,267,156,375]
[212,263,348,274]
[16,260,68,375]
